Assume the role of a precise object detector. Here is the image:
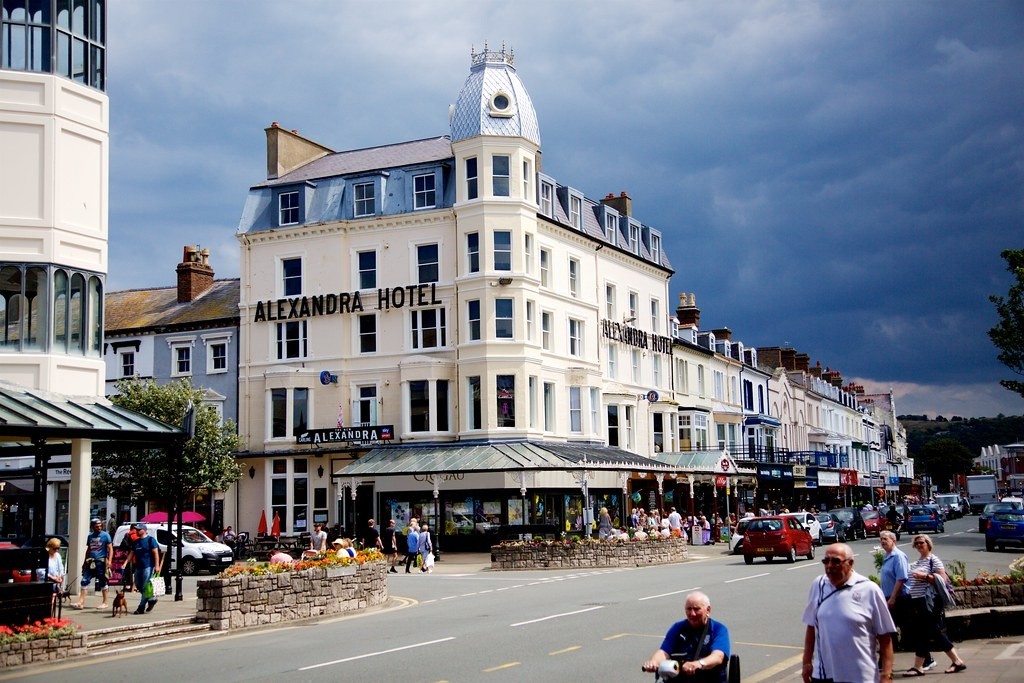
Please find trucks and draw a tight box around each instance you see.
[966,474,1000,516]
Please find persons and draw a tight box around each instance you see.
[70,519,113,610]
[119,523,140,593]
[121,523,160,615]
[607,525,629,541]
[399,514,433,574]
[35,538,65,614]
[215,525,245,557]
[107,512,116,542]
[599,507,613,530]
[642,592,730,683]
[901,533,968,676]
[802,543,897,683]
[383,520,398,573]
[310,522,327,554]
[362,519,384,551]
[321,521,328,533]
[877,531,937,673]
[266,549,293,565]
[331,537,357,559]
[885,502,904,533]
[630,506,736,544]
[744,500,817,518]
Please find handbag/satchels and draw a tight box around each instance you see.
[945,572,953,594]
[682,529,689,540]
[142,573,154,598]
[416,554,422,566]
[426,553,435,568]
[149,576,166,597]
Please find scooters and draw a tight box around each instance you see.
[885,517,904,540]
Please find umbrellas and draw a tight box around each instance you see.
[257,508,268,533]
[174,510,207,523]
[271,512,281,542]
[142,511,169,522]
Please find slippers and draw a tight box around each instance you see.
[945,662,967,674]
[903,667,926,677]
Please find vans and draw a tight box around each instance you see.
[113,521,235,576]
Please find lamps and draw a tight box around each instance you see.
[318,464,325,480]
[500,278,512,284]
[349,452,359,459]
[249,466,256,480]
[624,317,637,322]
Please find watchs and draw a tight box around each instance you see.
[925,573,929,580]
[698,659,706,670]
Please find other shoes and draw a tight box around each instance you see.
[70,603,84,610]
[134,600,157,614]
[390,569,397,573]
[922,660,937,671]
[96,603,109,609]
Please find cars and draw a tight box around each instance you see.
[813,513,849,544]
[778,511,824,547]
[860,510,892,540]
[985,509,1024,552]
[827,507,869,541]
[977,498,1024,534]
[743,514,815,563]
[730,517,755,556]
[880,494,969,535]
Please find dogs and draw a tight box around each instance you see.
[56,584,73,603]
[111,589,129,618]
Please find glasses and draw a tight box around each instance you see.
[52,547,60,550]
[914,541,925,545]
[334,543,339,545]
[822,557,841,564]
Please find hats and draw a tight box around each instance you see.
[90,518,101,528]
[313,523,322,527]
[135,523,148,531]
[331,538,345,548]
[619,526,626,531]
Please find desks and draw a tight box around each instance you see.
[257,539,298,552]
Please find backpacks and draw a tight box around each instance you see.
[119,532,135,552]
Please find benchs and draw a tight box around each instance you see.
[254,550,293,561]
